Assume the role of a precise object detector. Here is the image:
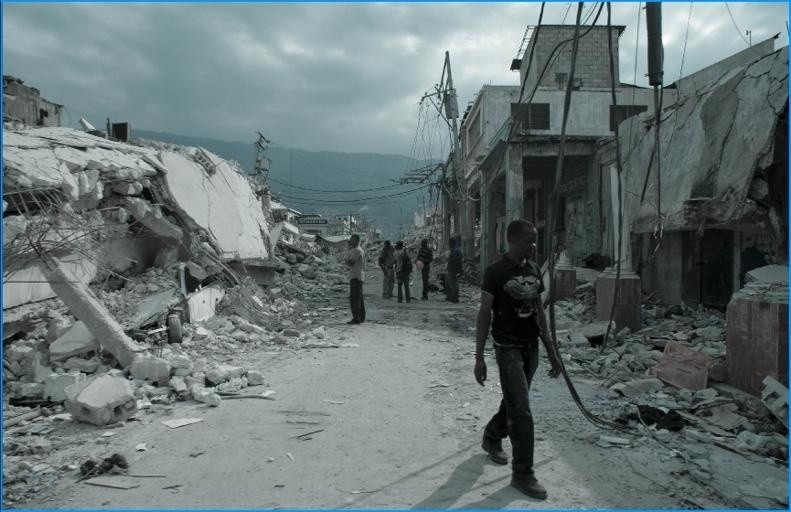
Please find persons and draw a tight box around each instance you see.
[444,237,465,304]
[378,239,395,299]
[392,240,413,303]
[344,234,367,325]
[474,217,563,501]
[414,238,434,301]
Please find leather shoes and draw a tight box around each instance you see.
[481,443,508,465]
[510,479,547,499]
